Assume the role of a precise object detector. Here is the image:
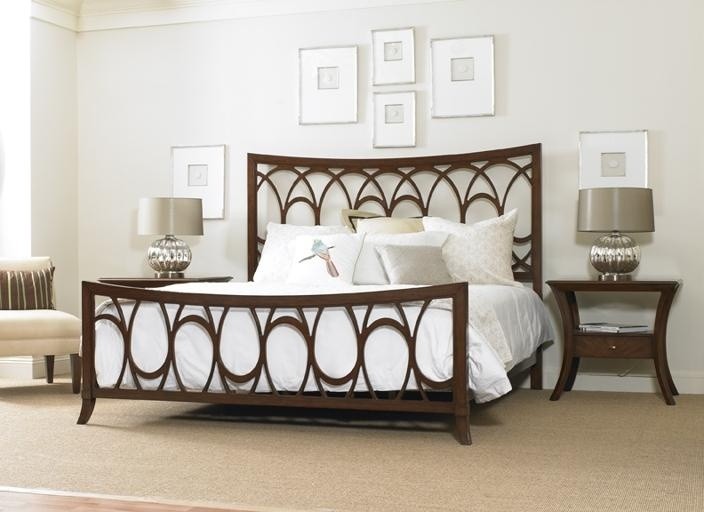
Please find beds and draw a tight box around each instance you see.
[77,142,554,447]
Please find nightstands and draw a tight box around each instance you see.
[101,273,235,291]
[541,275,683,405]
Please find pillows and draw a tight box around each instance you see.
[0,265,59,311]
[249,207,522,290]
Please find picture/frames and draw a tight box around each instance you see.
[369,89,419,151]
[295,43,362,127]
[168,144,228,220]
[576,128,650,192]
[367,26,417,88]
[426,33,497,121]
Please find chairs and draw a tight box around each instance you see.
[0,252,83,397]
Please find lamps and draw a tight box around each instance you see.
[135,197,206,281]
[574,186,660,282]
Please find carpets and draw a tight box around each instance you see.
[0,372,704,512]
